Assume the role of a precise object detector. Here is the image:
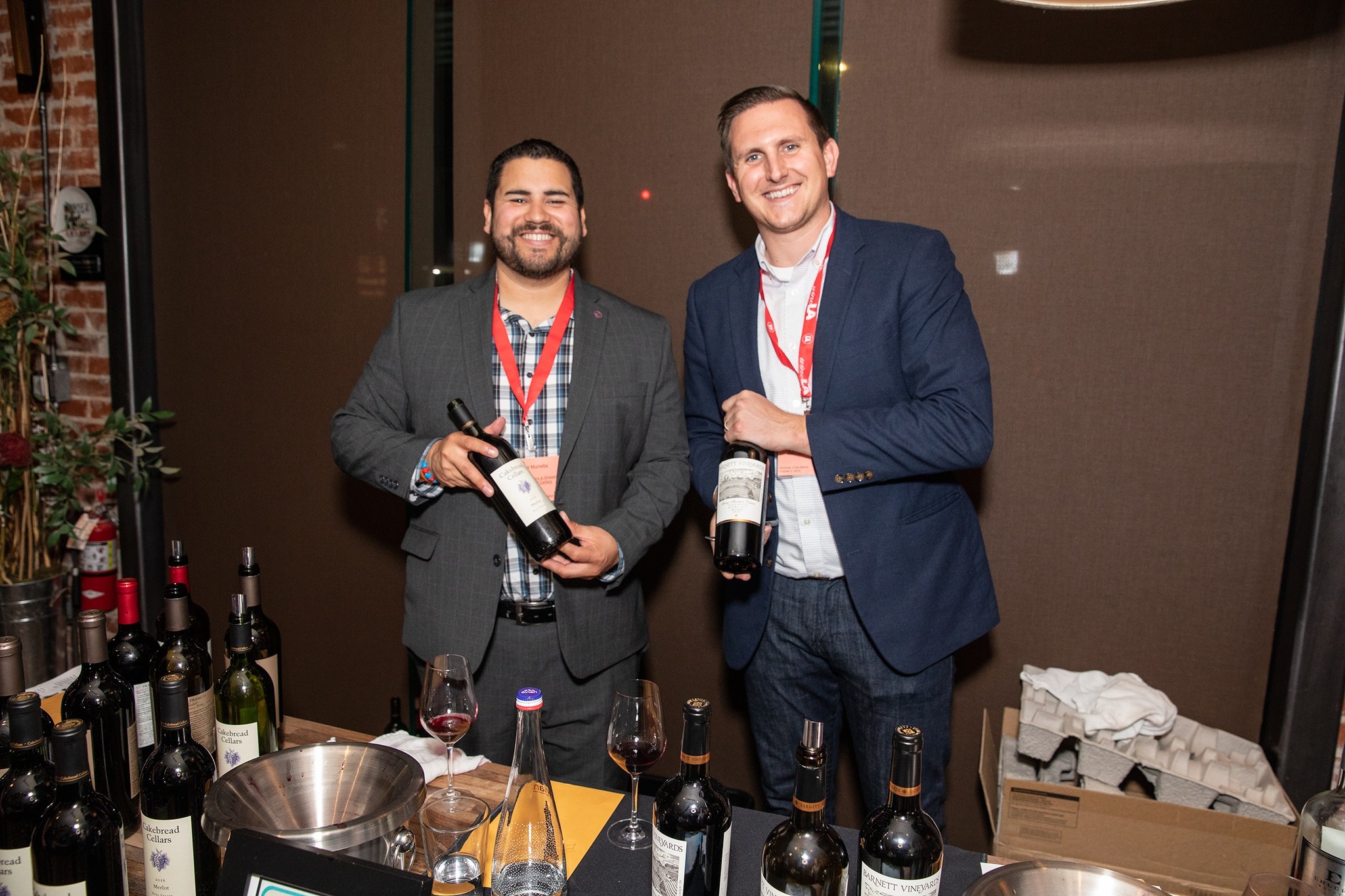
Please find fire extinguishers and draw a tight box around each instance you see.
[64,488,119,614]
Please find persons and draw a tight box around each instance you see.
[327,136,695,792]
[676,83,999,830]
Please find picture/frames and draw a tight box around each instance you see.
[213,828,433,896]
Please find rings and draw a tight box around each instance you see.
[724,419,729,431]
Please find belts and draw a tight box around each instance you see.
[496,600,556,627]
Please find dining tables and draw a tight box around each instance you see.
[125,716,987,896]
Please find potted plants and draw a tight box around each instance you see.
[1,33,180,691]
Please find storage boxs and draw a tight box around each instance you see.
[977,707,1302,896]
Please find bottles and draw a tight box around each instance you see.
[652,698,732,896]
[486,685,566,896]
[856,723,944,896]
[1292,745,1345,896]
[715,442,770,571]
[446,398,574,563]
[4,535,284,896]
[760,718,850,896]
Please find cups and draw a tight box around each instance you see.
[419,794,490,896]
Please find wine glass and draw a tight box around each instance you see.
[606,680,667,851]
[419,654,478,813]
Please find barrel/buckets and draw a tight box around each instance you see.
[957,859,1168,896]
[202,741,426,873]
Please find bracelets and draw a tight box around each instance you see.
[420,462,438,487]
